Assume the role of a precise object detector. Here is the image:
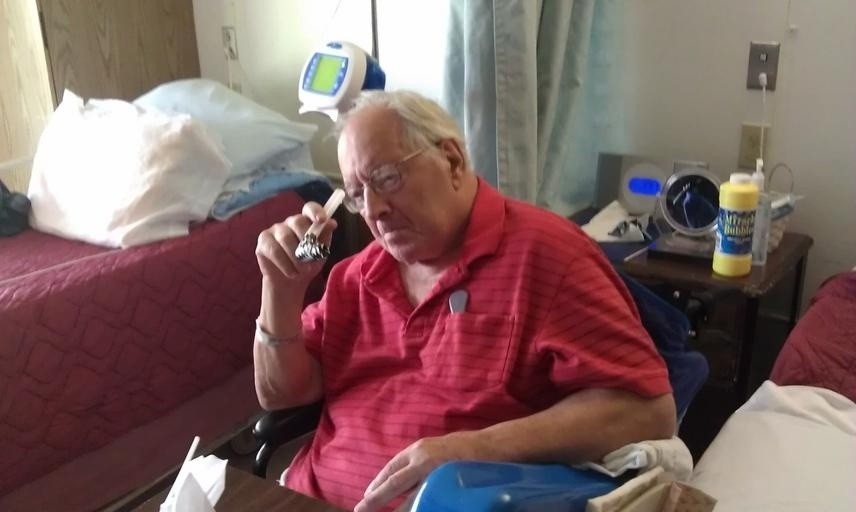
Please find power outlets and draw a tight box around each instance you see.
[747,41,780,90]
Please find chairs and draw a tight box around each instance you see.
[252,269,711,511]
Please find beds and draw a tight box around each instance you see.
[0,173,361,510]
[669,272,855,511]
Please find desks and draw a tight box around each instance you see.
[567,205,814,410]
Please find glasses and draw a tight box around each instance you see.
[343,144,428,215]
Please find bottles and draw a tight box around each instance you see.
[746,173,771,267]
[710,172,759,279]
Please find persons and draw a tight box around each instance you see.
[248,88,681,512]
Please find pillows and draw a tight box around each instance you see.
[27,88,234,250]
[130,78,317,223]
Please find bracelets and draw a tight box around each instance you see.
[252,316,303,346]
